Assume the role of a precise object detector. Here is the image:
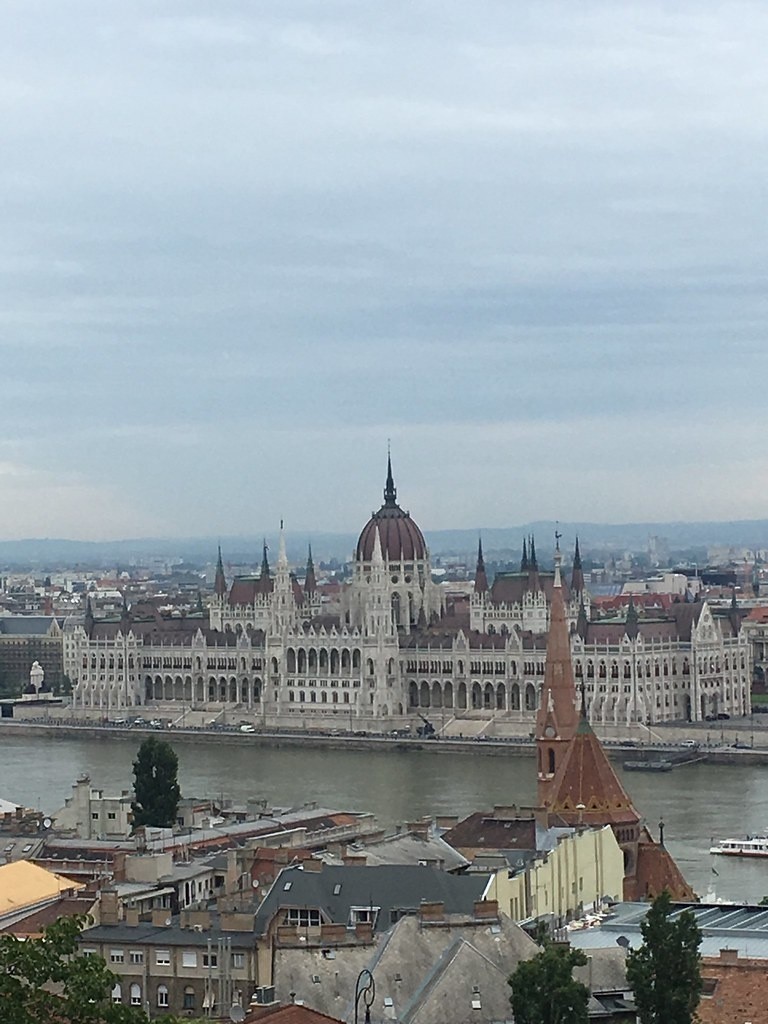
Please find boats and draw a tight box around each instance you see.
[710,838,768,858]
